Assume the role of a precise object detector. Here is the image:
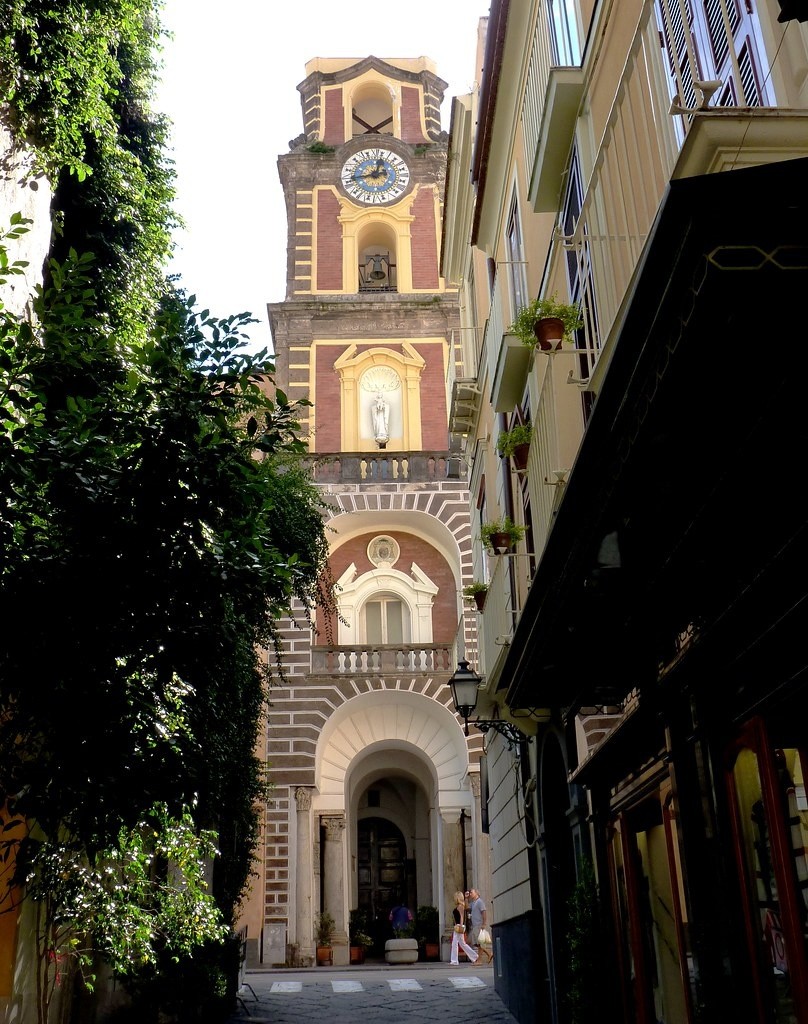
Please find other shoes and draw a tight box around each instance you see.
[487,955,493,965]
[471,960,483,966]
[445,960,460,966]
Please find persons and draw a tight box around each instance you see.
[389,896,413,938]
[465,887,493,966]
[371,393,390,444]
[445,891,479,965]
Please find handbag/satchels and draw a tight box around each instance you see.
[454,924,466,933]
[477,928,492,944]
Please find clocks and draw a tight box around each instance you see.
[338,143,417,205]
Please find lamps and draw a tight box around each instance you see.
[444,654,533,752]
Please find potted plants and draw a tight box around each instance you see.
[480,512,528,556]
[464,578,492,612]
[417,905,443,957]
[350,908,374,962]
[314,911,337,961]
[384,908,418,965]
[497,417,530,480]
[514,287,585,353]
